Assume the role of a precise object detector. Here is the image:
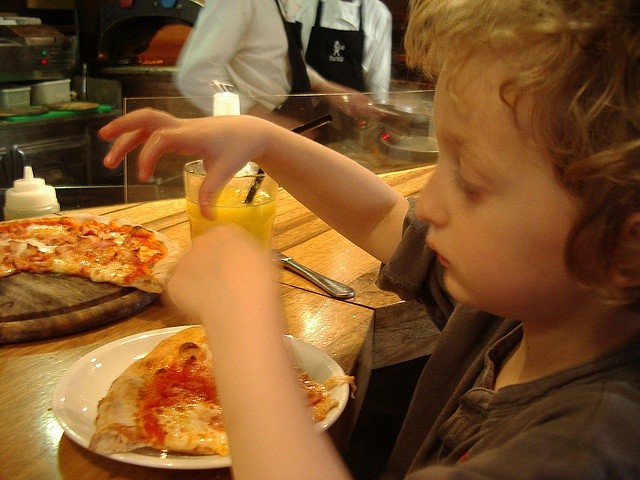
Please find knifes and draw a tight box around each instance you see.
[270,247,355,299]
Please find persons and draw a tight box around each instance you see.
[98,1,639,475]
[174,0,392,122]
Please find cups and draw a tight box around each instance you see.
[182,159,279,265]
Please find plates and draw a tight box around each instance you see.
[51,325,350,469]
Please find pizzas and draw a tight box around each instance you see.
[90,325,358,456]
[0,213,188,294]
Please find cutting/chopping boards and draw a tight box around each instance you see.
[1,214,180,345]
